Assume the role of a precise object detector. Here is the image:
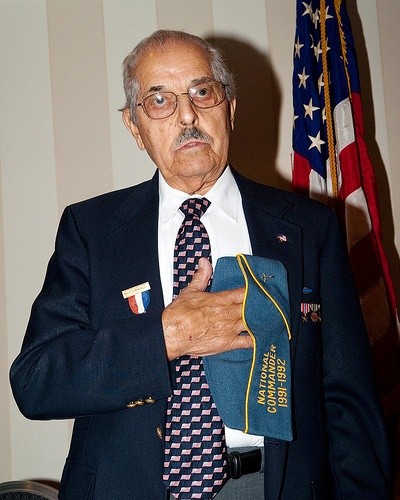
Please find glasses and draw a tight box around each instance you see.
[136,81,229,120]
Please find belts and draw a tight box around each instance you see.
[219,447,262,480]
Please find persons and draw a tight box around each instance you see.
[9,30,383,500]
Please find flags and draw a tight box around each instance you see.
[291,1,399,321]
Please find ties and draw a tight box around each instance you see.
[163,197,228,500]
